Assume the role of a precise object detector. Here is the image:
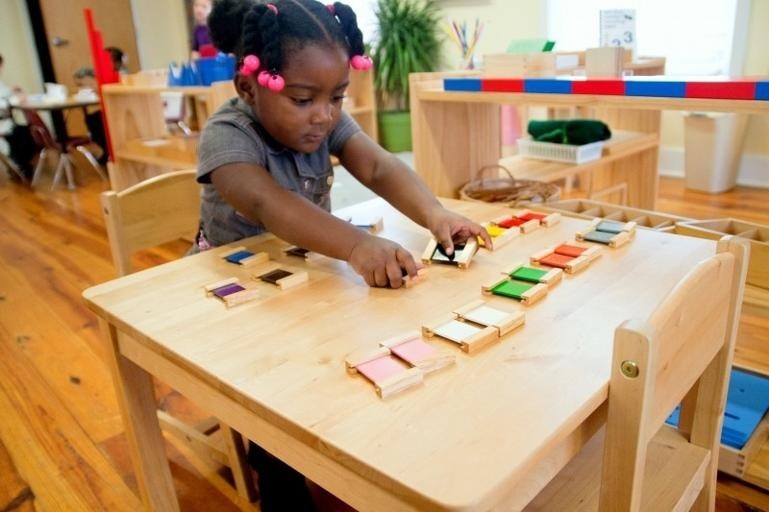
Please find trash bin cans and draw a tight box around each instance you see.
[683,111,750,193]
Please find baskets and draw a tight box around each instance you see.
[461,163,560,207]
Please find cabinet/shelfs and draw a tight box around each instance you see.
[91,72,241,196]
[483,45,665,213]
[505,123,664,217]
[677,218,768,291]
[536,200,696,236]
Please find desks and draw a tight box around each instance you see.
[0,82,103,185]
[403,68,768,196]
[72,184,737,512]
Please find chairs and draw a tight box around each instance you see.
[94,151,269,511]
[20,102,114,197]
[524,226,757,512]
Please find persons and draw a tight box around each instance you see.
[74,47,128,165]
[189,0,221,61]
[186,0,493,512]
[0,55,36,182]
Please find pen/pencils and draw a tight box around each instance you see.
[450,18,484,70]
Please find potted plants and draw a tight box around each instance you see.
[368,0,452,157]
